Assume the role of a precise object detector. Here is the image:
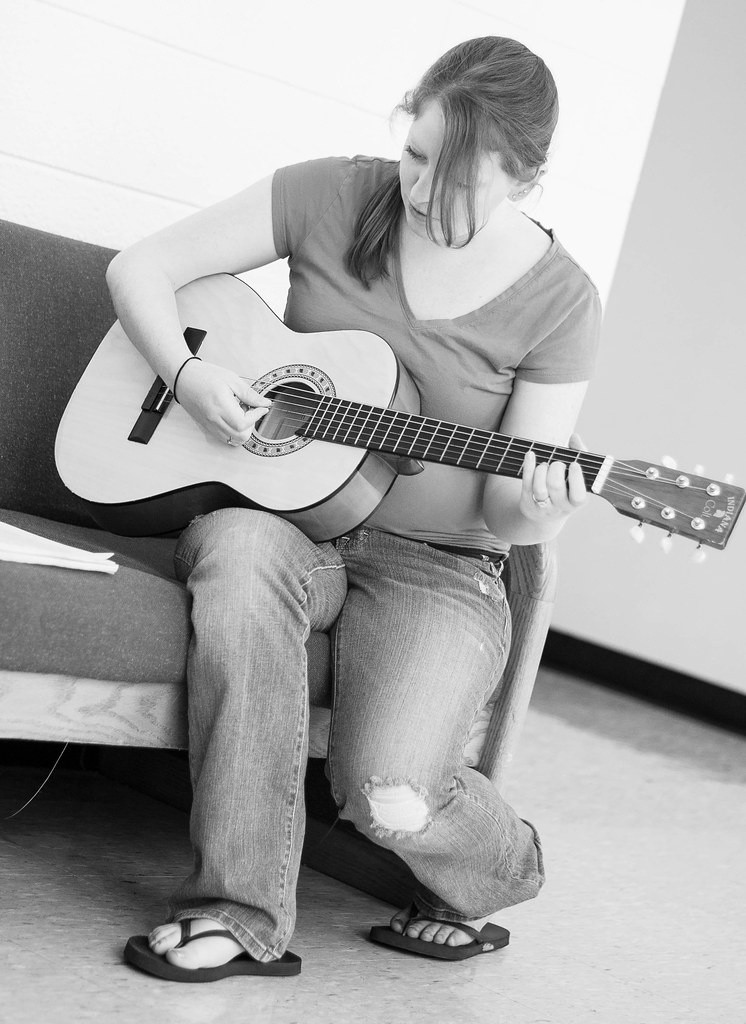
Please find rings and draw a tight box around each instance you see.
[532,494,549,509]
[226,436,233,445]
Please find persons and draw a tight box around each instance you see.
[104,33,603,984]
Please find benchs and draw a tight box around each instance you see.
[0,218,558,909]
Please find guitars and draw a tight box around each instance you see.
[51,270,746,555]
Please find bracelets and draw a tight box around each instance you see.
[173,356,202,404]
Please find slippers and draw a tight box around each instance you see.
[123,912,511,982]
